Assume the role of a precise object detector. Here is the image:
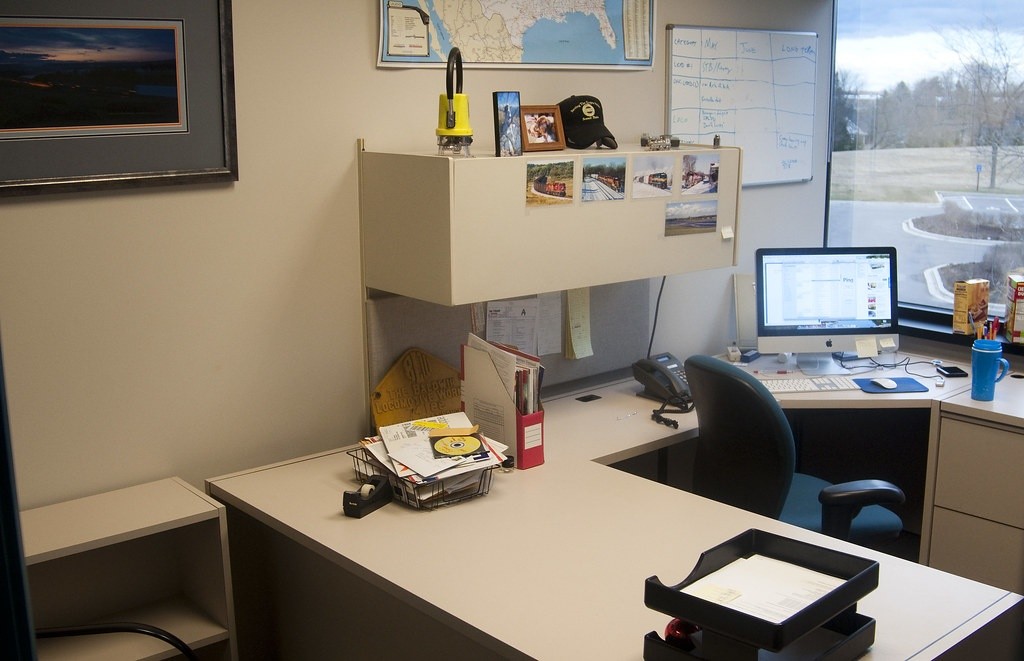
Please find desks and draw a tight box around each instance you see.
[201,348,1024,661]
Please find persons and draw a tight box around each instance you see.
[528,115,558,143]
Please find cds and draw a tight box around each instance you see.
[434,436,481,455]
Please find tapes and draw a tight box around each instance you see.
[360,484,375,497]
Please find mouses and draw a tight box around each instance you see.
[870,378,897,389]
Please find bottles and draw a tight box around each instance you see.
[640,133,720,151]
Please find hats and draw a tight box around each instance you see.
[558,95,618,149]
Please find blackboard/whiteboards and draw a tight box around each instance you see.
[665,24,820,187]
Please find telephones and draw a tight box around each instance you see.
[632,352,693,405]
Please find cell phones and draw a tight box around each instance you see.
[937,367,968,377]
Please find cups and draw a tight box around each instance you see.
[971,339,1009,401]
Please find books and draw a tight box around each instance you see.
[465,334,546,416]
[360,411,510,507]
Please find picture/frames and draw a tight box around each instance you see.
[0,0,244,199]
[521,101,567,152]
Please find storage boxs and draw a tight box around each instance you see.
[643,610,877,661]
[952,276,990,335]
[1005,273,1024,344]
[643,529,881,653]
[457,340,552,472]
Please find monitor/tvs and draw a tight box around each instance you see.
[757,248,900,376]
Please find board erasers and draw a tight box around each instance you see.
[741,350,760,363]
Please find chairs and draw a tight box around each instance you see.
[682,348,911,543]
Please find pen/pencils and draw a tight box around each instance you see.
[968,312,977,333]
[753,371,793,374]
[977,316,1000,340]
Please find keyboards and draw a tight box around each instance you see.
[758,377,861,394]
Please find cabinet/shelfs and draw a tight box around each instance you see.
[17,479,242,661]
[353,138,747,306]
[917,368,1024,597]
